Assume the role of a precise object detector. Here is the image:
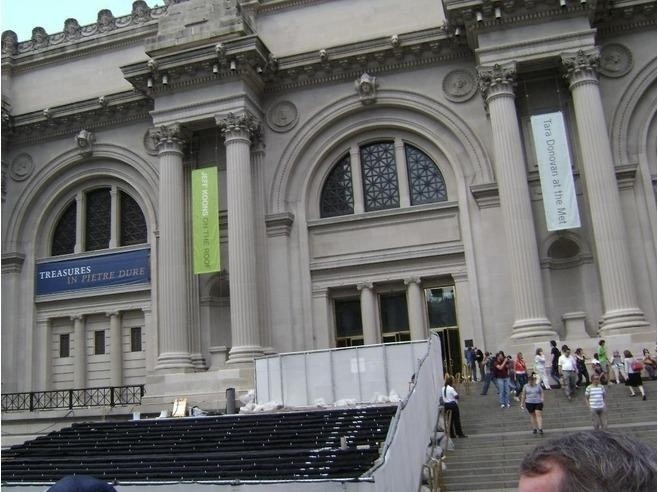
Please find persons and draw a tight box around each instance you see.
[44,473,117,492]
[583,372,609,428]
[516,427,657,491]
[440,374,469,438]
[456,338,657,410]
[518,375,545,436]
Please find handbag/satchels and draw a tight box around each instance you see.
[631,362,643,370]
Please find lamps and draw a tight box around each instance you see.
[449,0,591,39]
[77,129,88,149]
[359,70,372,95]
[144,61,265,88]
